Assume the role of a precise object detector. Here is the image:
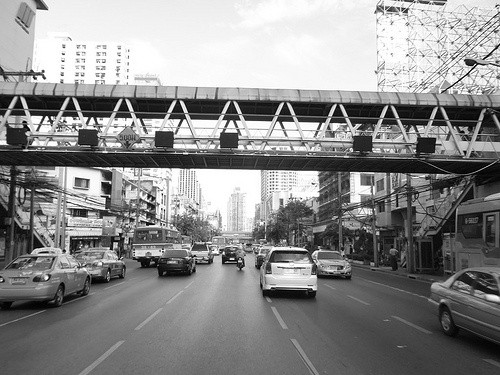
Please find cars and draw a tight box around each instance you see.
[0,254,91,308]
[311,249,352,279]
[259,246,318,298]
[431,266,500,348]
[157,249,196,276]
[74,247,126,283]
[182,235,288,269]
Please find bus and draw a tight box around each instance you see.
[454,193,500,276]
[132,225,182,268]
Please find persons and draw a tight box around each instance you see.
[459,130,469,156]
[467,125,480,157]
[340,244,354,258]
[389,245,398,270]
[21,120,32,134]
[235,245,246,268]
